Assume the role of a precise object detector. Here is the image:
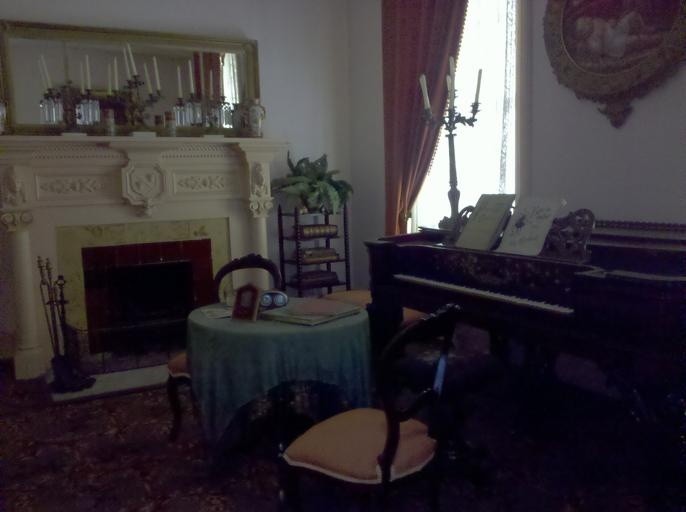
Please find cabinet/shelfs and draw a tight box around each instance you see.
[276,193,353,301]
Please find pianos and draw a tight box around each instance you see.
[368,212,686,502]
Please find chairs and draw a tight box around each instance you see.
[164,250,282,448]
[276,300,464,511]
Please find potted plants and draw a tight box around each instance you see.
[269,148,355,217]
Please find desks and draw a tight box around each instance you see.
[184,293,372,489]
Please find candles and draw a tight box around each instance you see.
[419,73,432,114]
[447,56,455,109]
[34,38,229,108]
[445,74,454,98]
[474,68,483,104]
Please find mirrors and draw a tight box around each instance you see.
[0,18,261,140]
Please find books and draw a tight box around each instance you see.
[258,298,361,326]
[495,196,562,257]
[455,193,516,253]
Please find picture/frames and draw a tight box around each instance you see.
[539,0,686,129]
